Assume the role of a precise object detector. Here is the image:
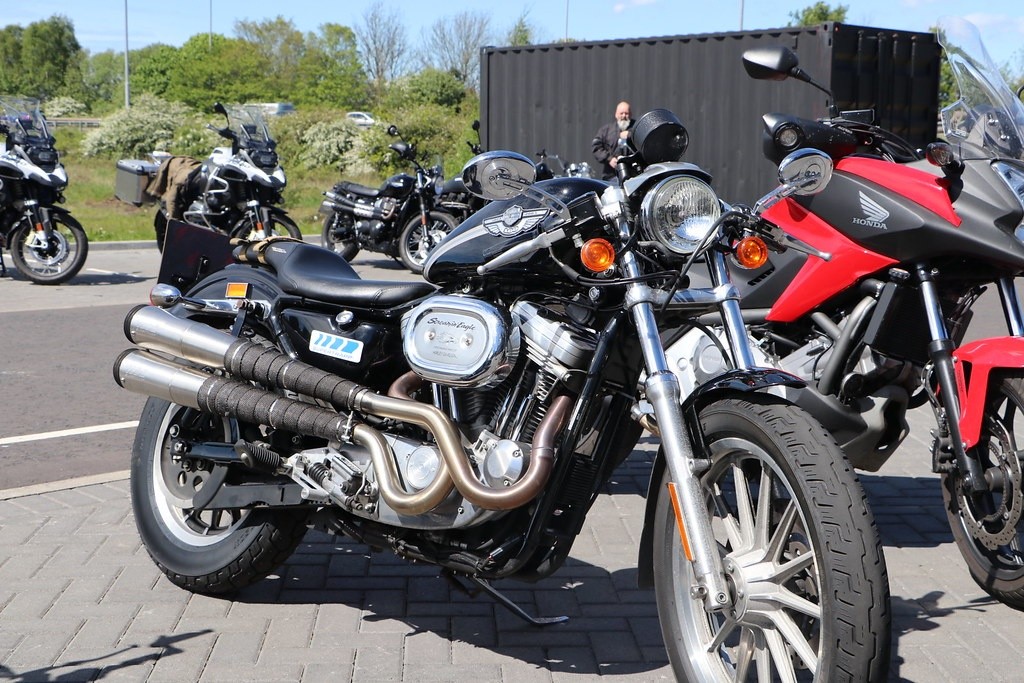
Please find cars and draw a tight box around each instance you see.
[347,110,382,129]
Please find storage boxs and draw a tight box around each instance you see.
[114,159,158,204]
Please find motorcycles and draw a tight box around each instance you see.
[317,124,477,275]
[0,100,91,285]
[623,24,1024,614]
[110,107,892,683]
[437,117,596,206]
[108,102,304,261]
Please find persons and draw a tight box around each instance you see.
[591,101,642,185]
[950,107,968,133]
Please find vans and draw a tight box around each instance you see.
[255,102,296,118]
